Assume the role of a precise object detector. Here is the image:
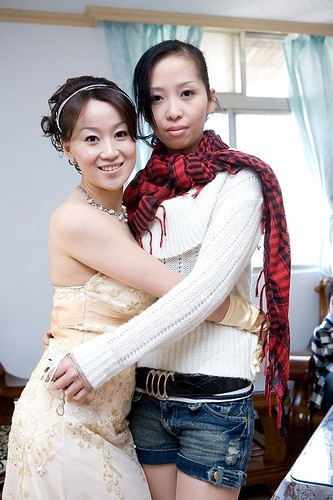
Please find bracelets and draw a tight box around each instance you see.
[67,355,92,394]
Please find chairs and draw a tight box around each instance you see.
[286,278,333,443]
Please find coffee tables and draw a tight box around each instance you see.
[242,389,293,483]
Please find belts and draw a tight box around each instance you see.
[134,367,251,400]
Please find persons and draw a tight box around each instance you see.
[42,39,266,500]
[1,75,273,499]
[307,283,333,414]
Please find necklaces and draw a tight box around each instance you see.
[73,185,130,224]
